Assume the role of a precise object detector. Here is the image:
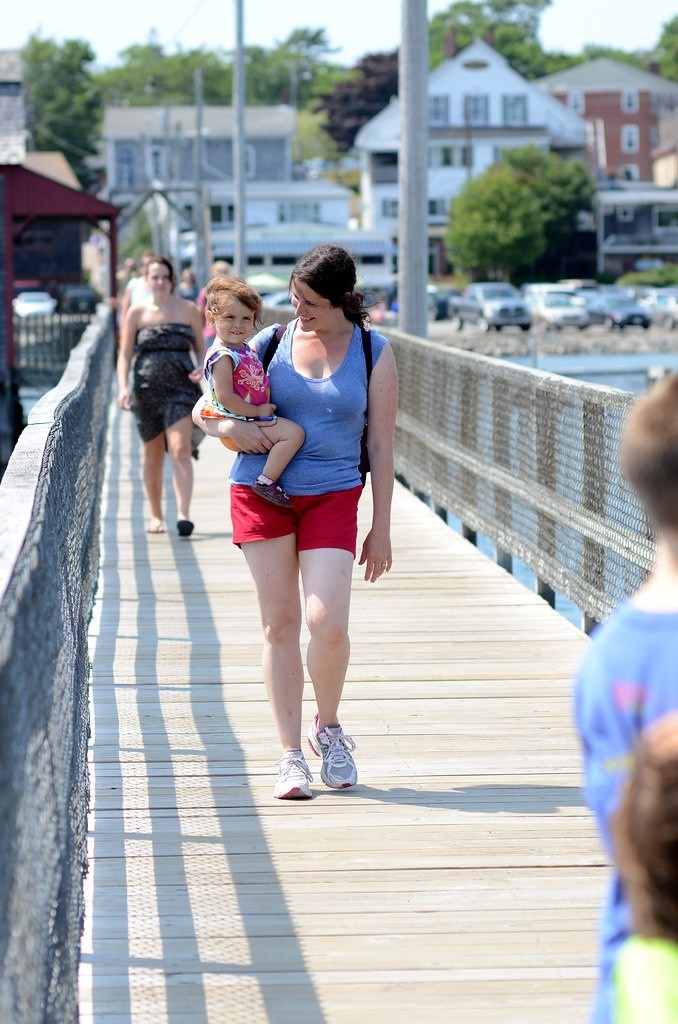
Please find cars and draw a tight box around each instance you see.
[260,277,678,332]
[12,285,99,319]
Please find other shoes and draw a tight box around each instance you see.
[250,479,293,507]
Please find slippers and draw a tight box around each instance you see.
[147,520,164,533]
[177,520,194,537]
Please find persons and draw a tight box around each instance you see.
[190,243,398,800]
[614,712,678,1023]
[572,370,678,1023]
[200,275,305,508]
[116,250,198,343]
[116,257,206,536]
[195,261,231,393]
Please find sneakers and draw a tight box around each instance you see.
[307,713,358,789]
[271,755,313,798]
